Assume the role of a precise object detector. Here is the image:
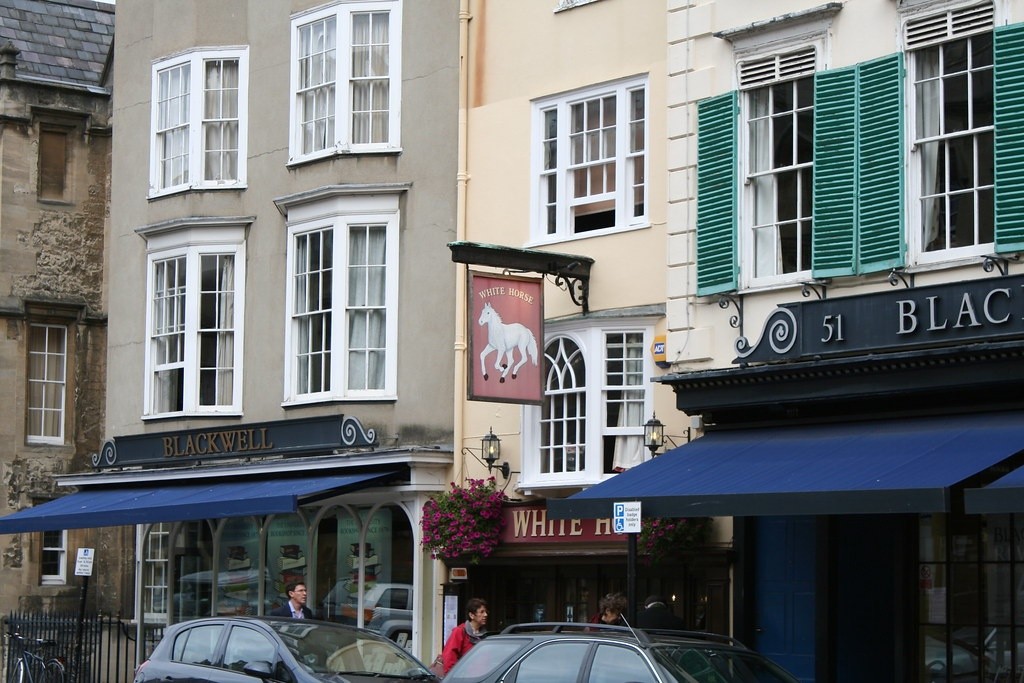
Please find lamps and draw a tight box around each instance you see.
[481,426,510,480]
[644,410,691,458]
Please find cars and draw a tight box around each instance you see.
[129,612,442,683]
[448,621,803,682]
[362,582,413,623]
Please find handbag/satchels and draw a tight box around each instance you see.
[429,654,445,678]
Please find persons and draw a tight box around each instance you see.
[269,582,314,620]
[442,598,488,674]
[584,593,684,634]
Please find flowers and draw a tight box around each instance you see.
[624,515,714,566]
[418,475,509,565]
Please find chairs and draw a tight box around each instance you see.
[300,643,327,666]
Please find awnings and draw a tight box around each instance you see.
[545,411,1024,521]
[0,466,405,535]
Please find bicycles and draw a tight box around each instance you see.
[5,631,66,683]
